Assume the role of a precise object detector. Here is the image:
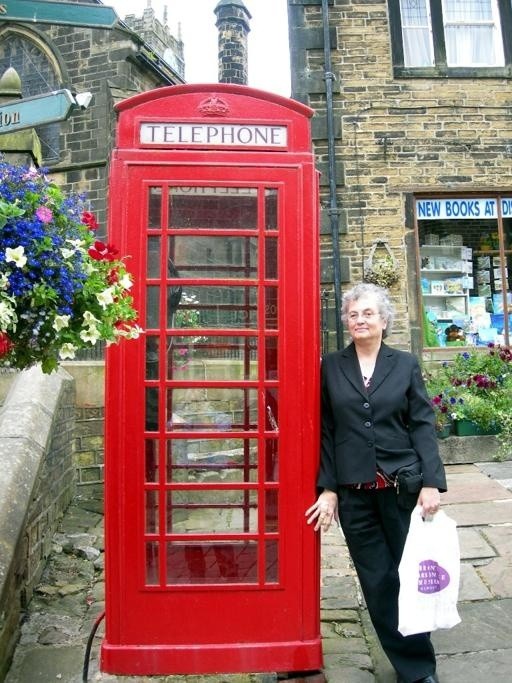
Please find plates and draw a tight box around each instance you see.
[419,244,470,321]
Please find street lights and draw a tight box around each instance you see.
[392,460,423,512]
[394,500,463,638]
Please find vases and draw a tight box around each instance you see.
[171,291,210,372]
[420,340,512,462]
[0,153,149,378]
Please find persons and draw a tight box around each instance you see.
[304,281,450,682]
[163,376,238,577]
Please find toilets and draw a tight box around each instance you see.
[147,250,182,328]
